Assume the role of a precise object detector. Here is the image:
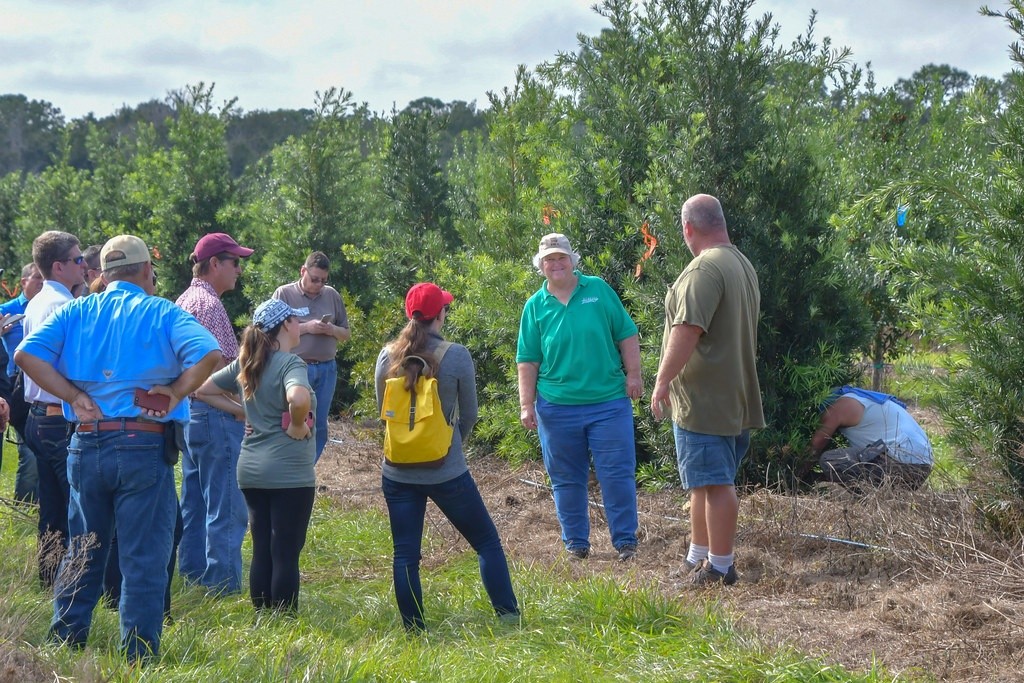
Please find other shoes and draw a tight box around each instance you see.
[618,542,637,566]
[669,556,738,591]
[560,545,588,565]
[666,555,700,583]
[499,608,525,630]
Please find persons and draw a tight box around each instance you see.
[175,233,255,603]
[800,386,934,495]
[15,235,222,669]
[374,282,528,641]
[0,337,12,432]
[195,299,318,628]
[651,194,766,591]
[22,230,89,592]
[515,233,644,562]
[272,250,351,465]
[0,262,43,509]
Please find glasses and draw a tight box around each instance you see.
[305,267,329,287]
[219,258,240,267]
[53,256,84,267]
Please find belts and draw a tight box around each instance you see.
[75,420,165,432]
[301,358,324,365]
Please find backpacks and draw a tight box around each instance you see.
[379,340,457,468]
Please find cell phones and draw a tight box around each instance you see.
[857,439,889,464]
[134,387,171,414]
[281,410,315,429]
[2,313,26,329]
[321,314,332,324]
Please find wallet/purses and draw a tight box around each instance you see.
[855,439,889,465]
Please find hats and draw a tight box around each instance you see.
[538,233,572,258]
[252,299,310,332]
[100,234,159,269]
[194,232,255,262]
[405,283,453,321]
